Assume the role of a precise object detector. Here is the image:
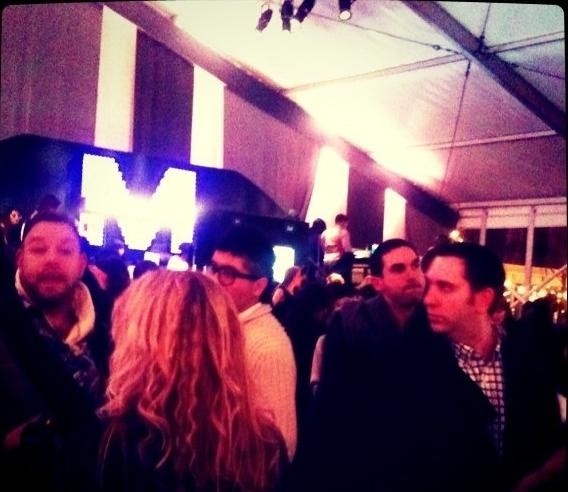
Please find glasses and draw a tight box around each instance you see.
[201,263,257,287]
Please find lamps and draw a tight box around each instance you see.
[256,0,351,33]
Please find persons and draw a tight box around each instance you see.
[1,215,567,491]
